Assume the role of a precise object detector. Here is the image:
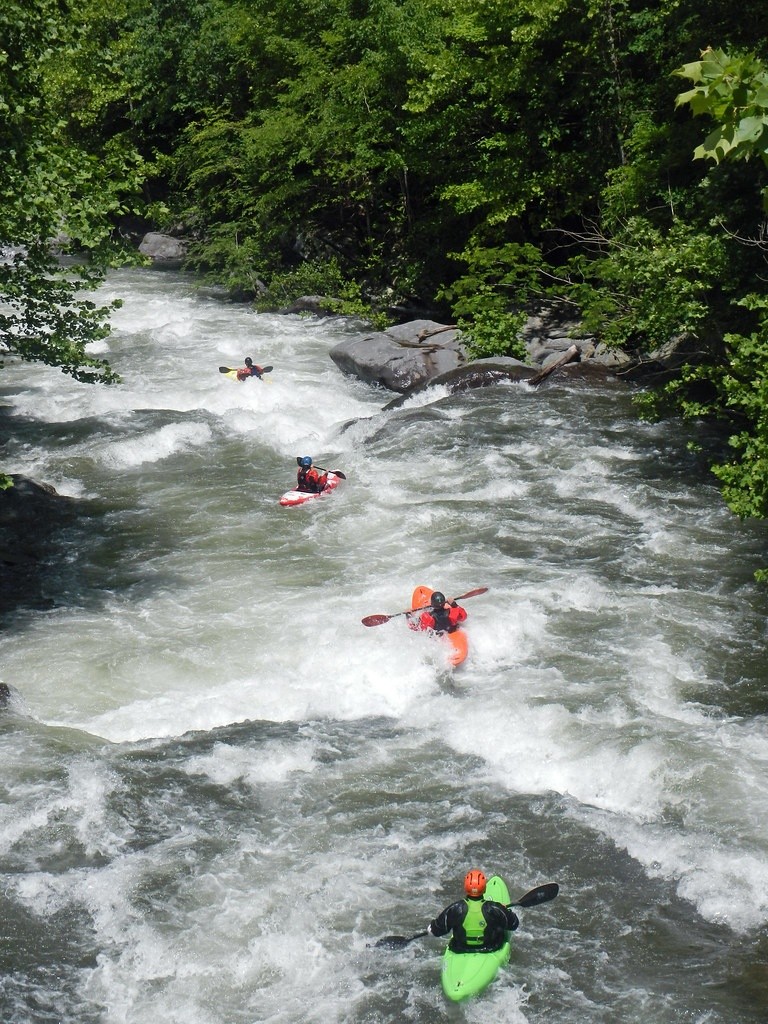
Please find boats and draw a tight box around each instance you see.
[226,367,268,384]
[408,585,470,670]
[443,873,515,1000]
[281,468,339,507]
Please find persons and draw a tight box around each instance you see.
[297,456,329,493]
[237,357,264,381]
[405,592,467,637]
[428,870,519,954]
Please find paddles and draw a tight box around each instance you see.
[296,456,347,481]
[373,882,560,951]
[218,365,274,373]
[360,587,489,628]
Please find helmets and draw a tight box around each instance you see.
[431,592,445,608]
[464,870,486,897]
[301,456,312,468]
[245,357,252,364]
